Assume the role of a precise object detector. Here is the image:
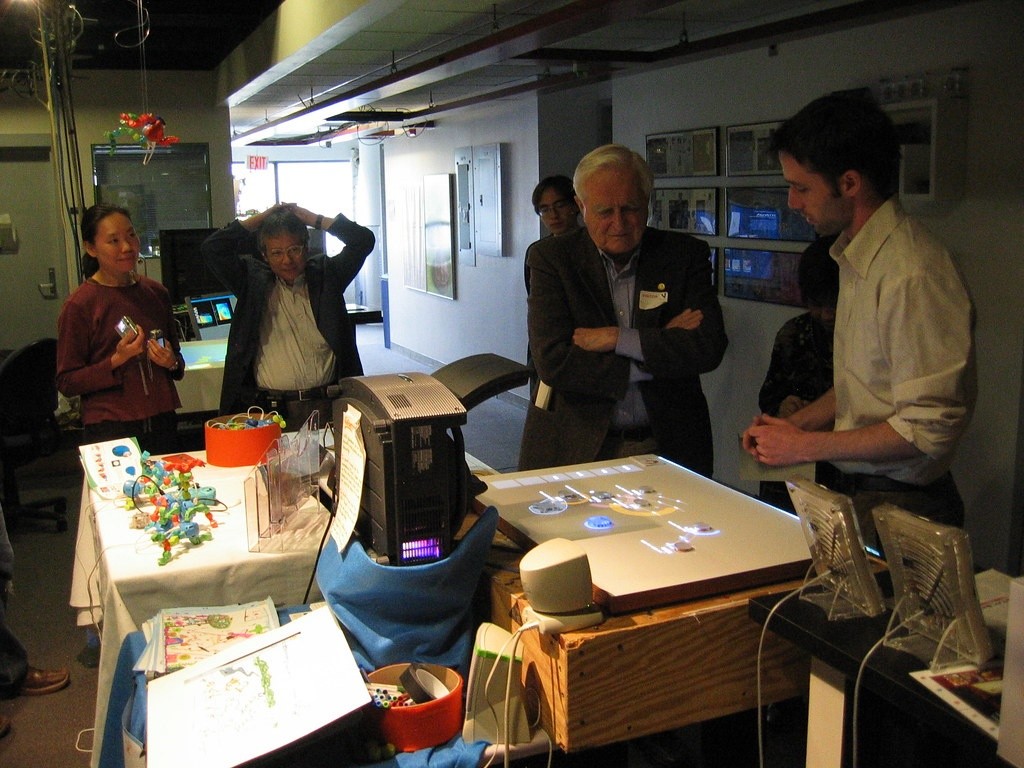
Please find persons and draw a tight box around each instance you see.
[518,143,729,479]
[201,201,375,432]
[57,204,186,454]
[742,90,978,598]
[673,192,686,229]
[758,235,840,418]
[0,501,70,737]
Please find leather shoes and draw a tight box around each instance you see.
[17,664,70,693]
[0,713,10,734]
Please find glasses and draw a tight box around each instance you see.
[270,243,304,262]
[538,201,572,218]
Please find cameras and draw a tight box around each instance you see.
[114,316,139,344]
[149,330,165,349]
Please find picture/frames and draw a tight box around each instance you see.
[725,187,817,243]
[724,247,808,308]
[643,127,720,180]
[646,187,719,237]
[726,118,793,178]
[423,173,455,300]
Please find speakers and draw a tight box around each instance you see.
[521,537,593,613]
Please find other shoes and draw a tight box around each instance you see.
[78,634,101,666]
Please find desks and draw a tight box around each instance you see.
[749,562,1024,767]
[173,337,230,413]
[71,450,336,768]
[454,510,808,753]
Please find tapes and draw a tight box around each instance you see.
[400,664,450,704]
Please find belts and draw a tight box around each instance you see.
[253,388,324,401]
[606,426,652,442]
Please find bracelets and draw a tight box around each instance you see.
[168,355,179,374]
[316,214,321,230]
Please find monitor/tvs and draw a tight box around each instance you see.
[185,289,238,341]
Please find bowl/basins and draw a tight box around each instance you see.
[205,413,282,467]
[365,663,463,752]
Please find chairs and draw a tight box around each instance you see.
[1,339,70,531]
